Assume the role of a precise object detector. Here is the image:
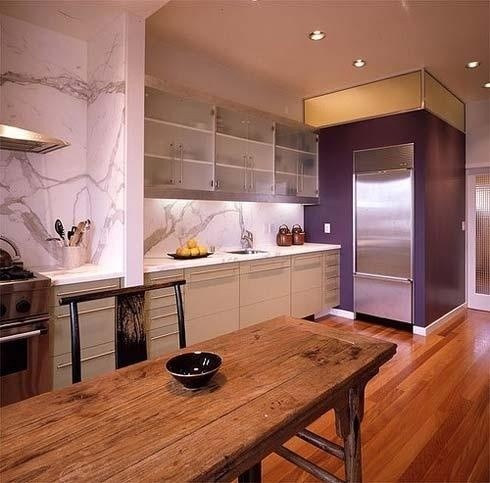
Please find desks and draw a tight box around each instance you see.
[0,315,397,483]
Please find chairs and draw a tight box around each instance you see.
[58,280,188,386]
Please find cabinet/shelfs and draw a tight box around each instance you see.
[52,277,125,392]
[144,249,340,360]
[145,72,321,206]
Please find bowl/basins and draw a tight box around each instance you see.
[164,350,223,390]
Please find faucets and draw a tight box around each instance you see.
[242,229,254,247]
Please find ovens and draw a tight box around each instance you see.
[0,280,52,408]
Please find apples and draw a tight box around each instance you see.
[176,240,207,256]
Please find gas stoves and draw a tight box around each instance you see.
[0,265,34,280]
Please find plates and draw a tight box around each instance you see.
[167,251,214,259]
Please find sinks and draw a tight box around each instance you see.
[226,249,267,255]
